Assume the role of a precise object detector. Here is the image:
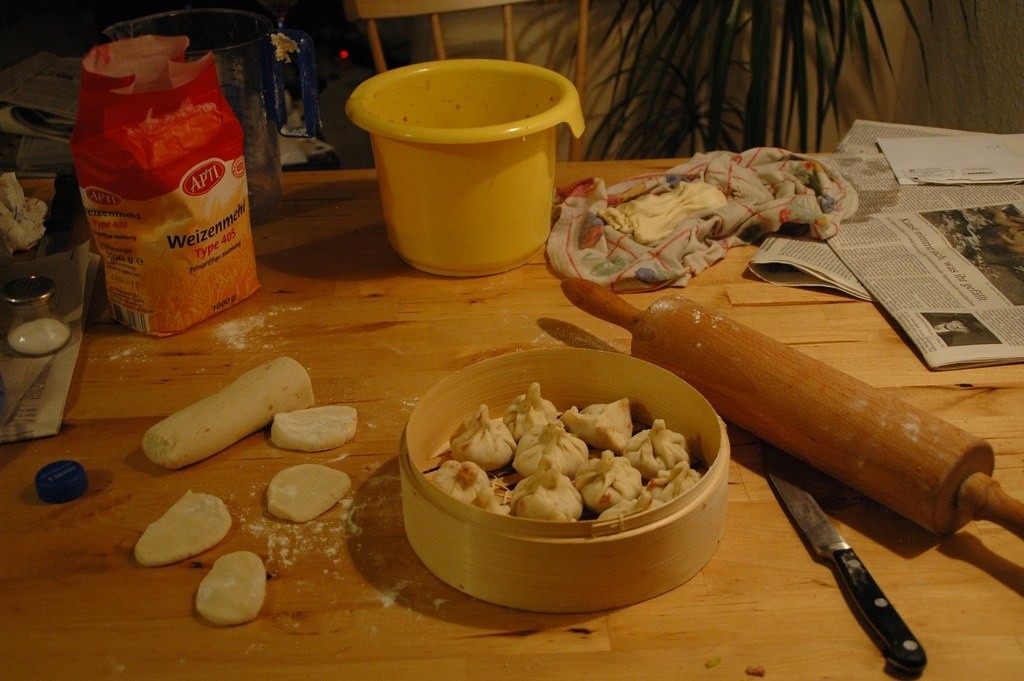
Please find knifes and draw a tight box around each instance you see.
[759,439,927,677]
[35,173,80,256]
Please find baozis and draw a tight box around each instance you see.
[559,397,633,456]
[596,490,666,521]
[429,460,492,511]
[510,454,584,521]
[572,449,642,515]
[645,460,710,503]
[449,403,518,471]
[512,419,589,481]
[622,418,693,480]
[502,382,557,441]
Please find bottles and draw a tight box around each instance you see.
[3,274,72,356]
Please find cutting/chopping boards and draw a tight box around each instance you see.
[0,158,1024,681]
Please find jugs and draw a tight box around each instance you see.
[100,7,321,228]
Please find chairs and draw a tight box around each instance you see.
[344,0,590,162]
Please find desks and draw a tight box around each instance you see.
[0,158,1024,681]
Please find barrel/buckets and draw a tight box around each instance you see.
[344,58,586,278]
